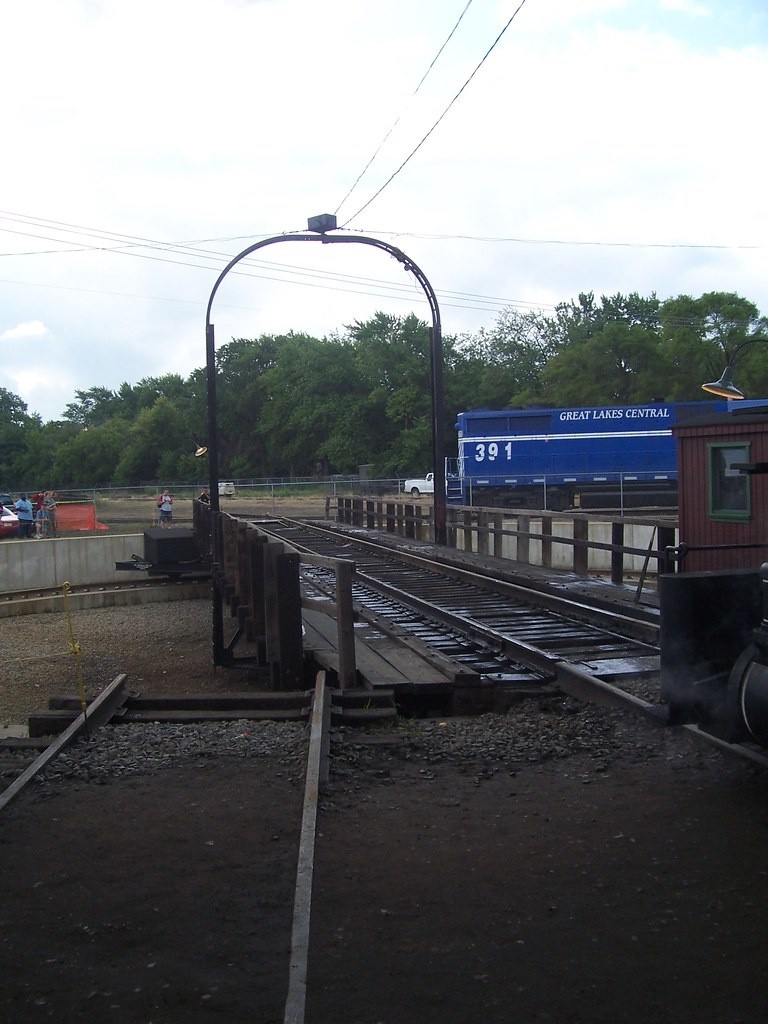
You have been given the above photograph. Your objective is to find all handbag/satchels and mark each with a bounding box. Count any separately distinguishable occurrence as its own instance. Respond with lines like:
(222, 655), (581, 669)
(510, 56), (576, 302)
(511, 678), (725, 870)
(157, 496), (162, 508)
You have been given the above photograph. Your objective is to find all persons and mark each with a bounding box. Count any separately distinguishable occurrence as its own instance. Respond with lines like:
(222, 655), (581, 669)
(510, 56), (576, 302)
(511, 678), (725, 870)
(157, 489), (173, 530)
(198, 487), (210, 505)
(32, 491), (60, 538)
(15, 493), (34, 539)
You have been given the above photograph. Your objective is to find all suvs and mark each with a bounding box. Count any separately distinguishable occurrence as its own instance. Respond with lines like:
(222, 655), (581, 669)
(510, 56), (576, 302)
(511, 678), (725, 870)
(217, 482), (236, 497)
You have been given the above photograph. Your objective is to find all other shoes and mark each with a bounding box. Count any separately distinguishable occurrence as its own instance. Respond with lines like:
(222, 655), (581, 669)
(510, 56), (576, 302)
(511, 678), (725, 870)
(168, 526), (171, 529)
(34, 534), (41, 539)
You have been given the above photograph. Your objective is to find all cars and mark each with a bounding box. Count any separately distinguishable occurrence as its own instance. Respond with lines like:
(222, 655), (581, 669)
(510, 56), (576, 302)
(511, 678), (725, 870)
(0, 491), (58, 539)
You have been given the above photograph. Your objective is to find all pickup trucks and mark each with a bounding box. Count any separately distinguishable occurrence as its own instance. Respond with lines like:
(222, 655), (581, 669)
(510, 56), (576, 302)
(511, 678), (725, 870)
(404, 472), (457, 497)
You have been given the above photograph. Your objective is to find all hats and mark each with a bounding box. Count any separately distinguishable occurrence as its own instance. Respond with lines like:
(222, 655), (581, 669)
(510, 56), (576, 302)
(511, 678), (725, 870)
(41, 502), (47, 506)
